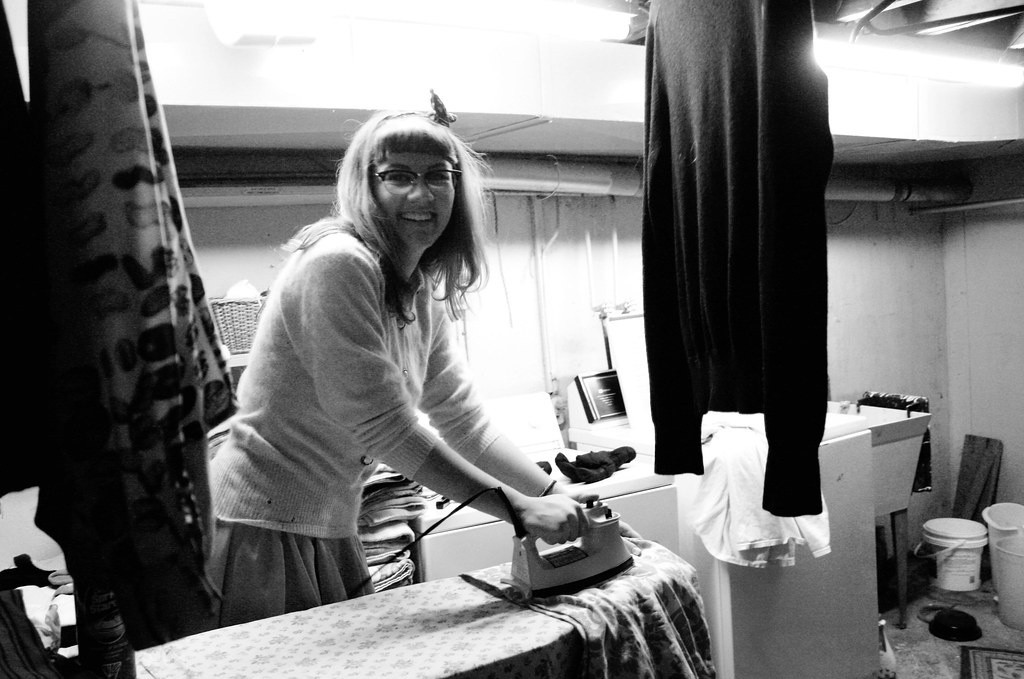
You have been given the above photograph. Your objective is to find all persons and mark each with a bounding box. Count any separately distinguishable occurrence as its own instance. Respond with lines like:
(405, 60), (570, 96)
(170, 107), (640, 638)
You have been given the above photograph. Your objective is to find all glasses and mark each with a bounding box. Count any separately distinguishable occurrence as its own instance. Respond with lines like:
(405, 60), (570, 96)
(374, 167), (462, 195)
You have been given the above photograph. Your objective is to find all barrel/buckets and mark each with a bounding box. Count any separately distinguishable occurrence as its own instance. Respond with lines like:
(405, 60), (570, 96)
(982, 502), (1024, 592)
(994, 536), (1024, 631)
(913, 518), (989, 591)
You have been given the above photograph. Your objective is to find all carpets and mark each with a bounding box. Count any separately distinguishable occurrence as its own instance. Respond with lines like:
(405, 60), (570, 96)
(959, 645), (1024, 679)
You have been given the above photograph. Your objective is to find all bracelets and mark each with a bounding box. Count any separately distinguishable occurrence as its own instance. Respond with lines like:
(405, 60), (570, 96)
(539, 479), (556, 498)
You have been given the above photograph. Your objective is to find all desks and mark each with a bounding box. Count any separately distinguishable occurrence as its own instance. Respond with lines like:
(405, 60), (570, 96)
(135, 534), (716, 679)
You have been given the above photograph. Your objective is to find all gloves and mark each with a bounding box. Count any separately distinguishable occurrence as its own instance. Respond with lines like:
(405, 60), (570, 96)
(555, 446), (636, 482)
